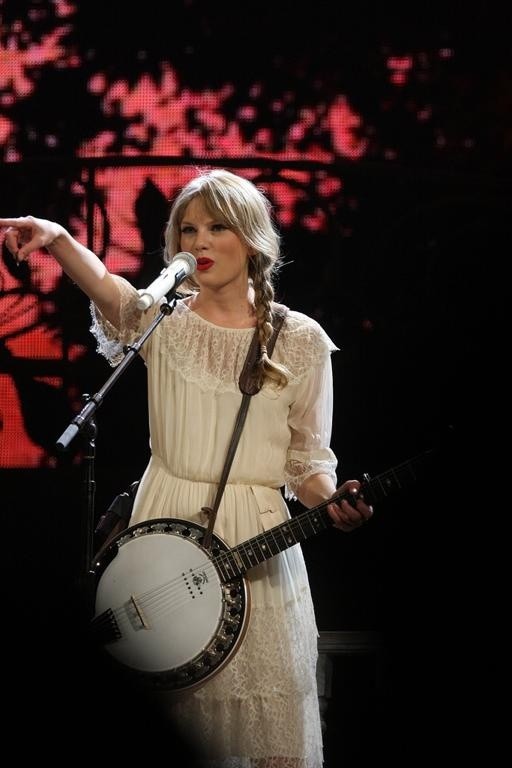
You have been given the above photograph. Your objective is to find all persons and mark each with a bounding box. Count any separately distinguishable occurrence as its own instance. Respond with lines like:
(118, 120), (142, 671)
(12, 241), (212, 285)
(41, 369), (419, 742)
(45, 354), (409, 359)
(0, 168), (376, 768)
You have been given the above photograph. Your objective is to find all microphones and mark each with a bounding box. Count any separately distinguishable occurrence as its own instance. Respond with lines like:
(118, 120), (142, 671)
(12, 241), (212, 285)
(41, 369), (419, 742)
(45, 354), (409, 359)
(137, 251), (197, 311)
